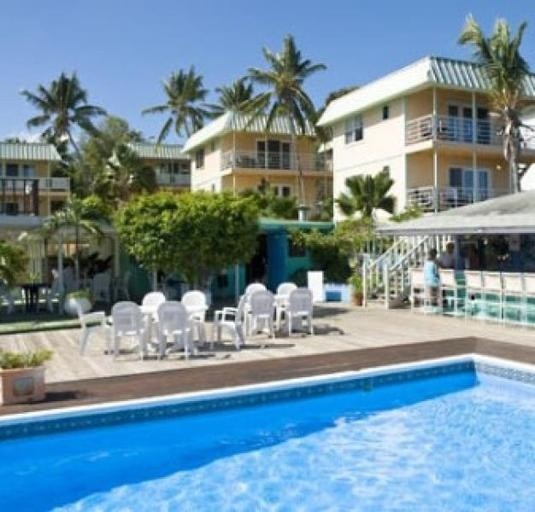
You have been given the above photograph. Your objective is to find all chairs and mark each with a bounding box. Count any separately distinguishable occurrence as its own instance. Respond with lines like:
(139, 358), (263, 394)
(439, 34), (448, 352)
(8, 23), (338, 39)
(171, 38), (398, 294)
(1, 278), (26, 315)
(68, 286), (208, 362)
(209, 280), (315, 352)
(37, 277), (64, 316)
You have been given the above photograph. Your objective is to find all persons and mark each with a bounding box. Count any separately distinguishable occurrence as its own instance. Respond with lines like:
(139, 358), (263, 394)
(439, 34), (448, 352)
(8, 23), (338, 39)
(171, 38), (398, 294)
(422, 237), (534, 308)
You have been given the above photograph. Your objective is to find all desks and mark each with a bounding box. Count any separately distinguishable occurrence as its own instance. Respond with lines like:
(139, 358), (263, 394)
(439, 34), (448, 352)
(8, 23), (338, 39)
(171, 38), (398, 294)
(16, 281), (48, 316)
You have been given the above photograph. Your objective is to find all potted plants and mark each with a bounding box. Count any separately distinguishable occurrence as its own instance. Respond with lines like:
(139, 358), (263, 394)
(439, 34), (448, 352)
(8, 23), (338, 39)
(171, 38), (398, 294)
(0, 344), (55, 405)
(349, 273), (364, 305)
